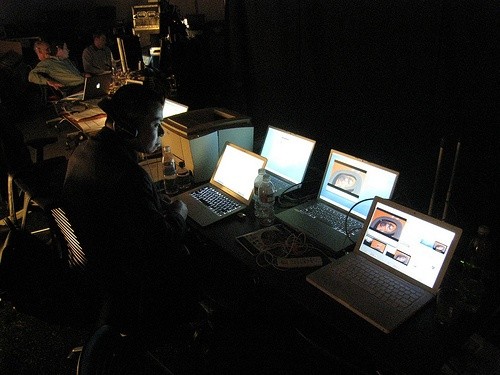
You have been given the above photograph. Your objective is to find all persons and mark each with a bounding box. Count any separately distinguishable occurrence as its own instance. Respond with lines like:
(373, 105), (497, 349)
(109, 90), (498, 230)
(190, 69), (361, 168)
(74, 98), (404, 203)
(60, 83), (189, 264)
(28, 40), (91, 90)
(82, 31), (112, 74)
(31, 41), (51, 69)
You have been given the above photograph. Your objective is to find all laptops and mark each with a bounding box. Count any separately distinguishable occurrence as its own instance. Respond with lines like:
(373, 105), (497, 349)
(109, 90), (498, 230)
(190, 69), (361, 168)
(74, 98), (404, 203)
(274, 148), (400, 253)
(66, 72), (112, 101)
(259, 125), (316, 200)
(170, 140), (268, 227)
(305, 195), (462, 335)
(162, 98), (189, 119)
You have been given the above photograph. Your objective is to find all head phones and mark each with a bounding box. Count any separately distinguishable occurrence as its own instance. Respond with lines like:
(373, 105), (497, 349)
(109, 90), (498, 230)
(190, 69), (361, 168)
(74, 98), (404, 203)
(98, 96), (139, 140)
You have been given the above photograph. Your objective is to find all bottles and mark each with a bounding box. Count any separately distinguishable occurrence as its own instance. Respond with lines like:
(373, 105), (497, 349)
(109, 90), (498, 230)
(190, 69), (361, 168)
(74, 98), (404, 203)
(164, 160), (179, 195)
(176, 160), (191, 191)
(253, 168), (267, 217)
(161, 145), (175, 173)
(464, 226), (490, 273)
(258, 174), (276, 226)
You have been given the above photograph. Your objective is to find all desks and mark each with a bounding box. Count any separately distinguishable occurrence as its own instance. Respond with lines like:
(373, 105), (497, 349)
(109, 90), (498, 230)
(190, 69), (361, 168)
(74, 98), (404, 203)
(56, 88), (500, 373)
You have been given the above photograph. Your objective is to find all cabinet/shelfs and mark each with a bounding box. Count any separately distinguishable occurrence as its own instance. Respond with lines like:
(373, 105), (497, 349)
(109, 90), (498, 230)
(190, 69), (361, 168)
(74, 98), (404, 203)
(130, 0), (163, 36)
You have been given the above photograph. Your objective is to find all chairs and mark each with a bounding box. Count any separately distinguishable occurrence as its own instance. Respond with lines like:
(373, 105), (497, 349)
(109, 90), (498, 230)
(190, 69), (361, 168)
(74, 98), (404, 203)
(8, 54), (69, 124)
(0, 125), (70, 236)
